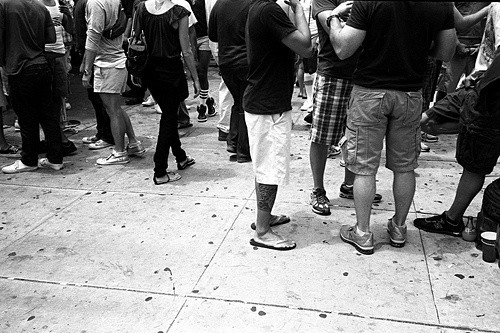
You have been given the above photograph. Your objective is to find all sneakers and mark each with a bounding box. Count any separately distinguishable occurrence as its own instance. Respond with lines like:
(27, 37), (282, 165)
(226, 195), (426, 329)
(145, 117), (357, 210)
(339, 182), (382, 202)
(310, 187), (331, 216)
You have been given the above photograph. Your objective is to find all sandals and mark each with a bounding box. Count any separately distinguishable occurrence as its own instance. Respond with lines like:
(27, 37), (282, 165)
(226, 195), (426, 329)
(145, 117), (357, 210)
(176, 156), (195, 170)
(153, 171), (182, 184)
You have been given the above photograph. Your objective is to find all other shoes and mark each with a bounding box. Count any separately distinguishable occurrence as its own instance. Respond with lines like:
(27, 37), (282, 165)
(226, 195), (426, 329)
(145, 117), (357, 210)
(205, 97), (218, 116)
(96, 149), (130, 165)
(88, 140), (113, 149)
(339, 224), (375, 255)
(327, 146), (342, 158)
(414, 211), (465, 238)
(420, 142), (430, 152)
(141, 95), (156, 106)
(38, 158), (64, 170)
(2, 160), (38, 173)
(197, 104), (208, 122)
(126, 140), (145, 154)
(177, 123), (194, 135)
(387, 215), (407, 248)
(420, 131), (437, 143)
(81, 135), (97, 143)
(0, 145), (22, 157)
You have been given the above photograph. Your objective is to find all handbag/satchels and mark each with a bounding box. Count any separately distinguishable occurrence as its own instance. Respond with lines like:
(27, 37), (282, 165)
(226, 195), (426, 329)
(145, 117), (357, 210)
(102, 6), (128, 41)
(127, 31), (149, 90)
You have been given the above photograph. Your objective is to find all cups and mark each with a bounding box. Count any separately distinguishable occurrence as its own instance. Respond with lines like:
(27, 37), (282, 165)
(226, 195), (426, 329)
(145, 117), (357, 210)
(481, 230), (498, 264)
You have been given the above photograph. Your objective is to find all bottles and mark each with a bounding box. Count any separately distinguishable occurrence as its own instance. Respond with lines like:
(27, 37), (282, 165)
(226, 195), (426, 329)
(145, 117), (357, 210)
(462, 216), (476, 242)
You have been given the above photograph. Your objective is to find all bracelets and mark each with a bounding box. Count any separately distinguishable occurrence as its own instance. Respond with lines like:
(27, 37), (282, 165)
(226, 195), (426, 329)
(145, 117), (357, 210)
(84, 70), (89, 76)
(326, 15), (340, 27)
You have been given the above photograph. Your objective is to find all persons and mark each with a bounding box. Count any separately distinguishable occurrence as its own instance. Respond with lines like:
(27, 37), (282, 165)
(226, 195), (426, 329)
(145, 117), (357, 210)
(208, 0), (500, 255)
(0, 0), (217, 185)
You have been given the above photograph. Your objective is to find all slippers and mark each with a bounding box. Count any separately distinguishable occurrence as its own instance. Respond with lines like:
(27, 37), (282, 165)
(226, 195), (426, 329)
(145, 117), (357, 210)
(251, 214), (290, 230)
(249, 233), (297, 251)
